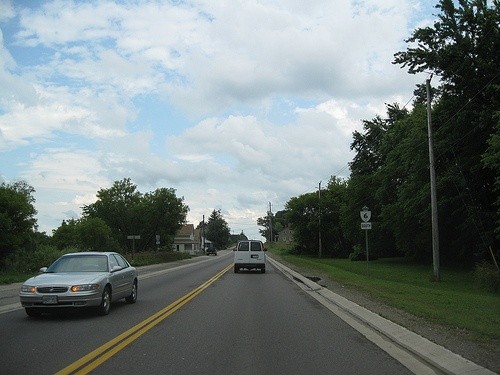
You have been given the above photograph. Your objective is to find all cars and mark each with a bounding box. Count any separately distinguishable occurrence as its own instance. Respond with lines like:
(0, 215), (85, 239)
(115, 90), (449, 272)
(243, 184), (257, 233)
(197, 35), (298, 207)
(19, 252), (139, 316)
(206, 247), (217, 256)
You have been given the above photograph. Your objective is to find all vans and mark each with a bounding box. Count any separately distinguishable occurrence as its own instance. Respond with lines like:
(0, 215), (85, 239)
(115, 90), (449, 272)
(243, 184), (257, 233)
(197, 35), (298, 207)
(233, 240), (268, 274)
